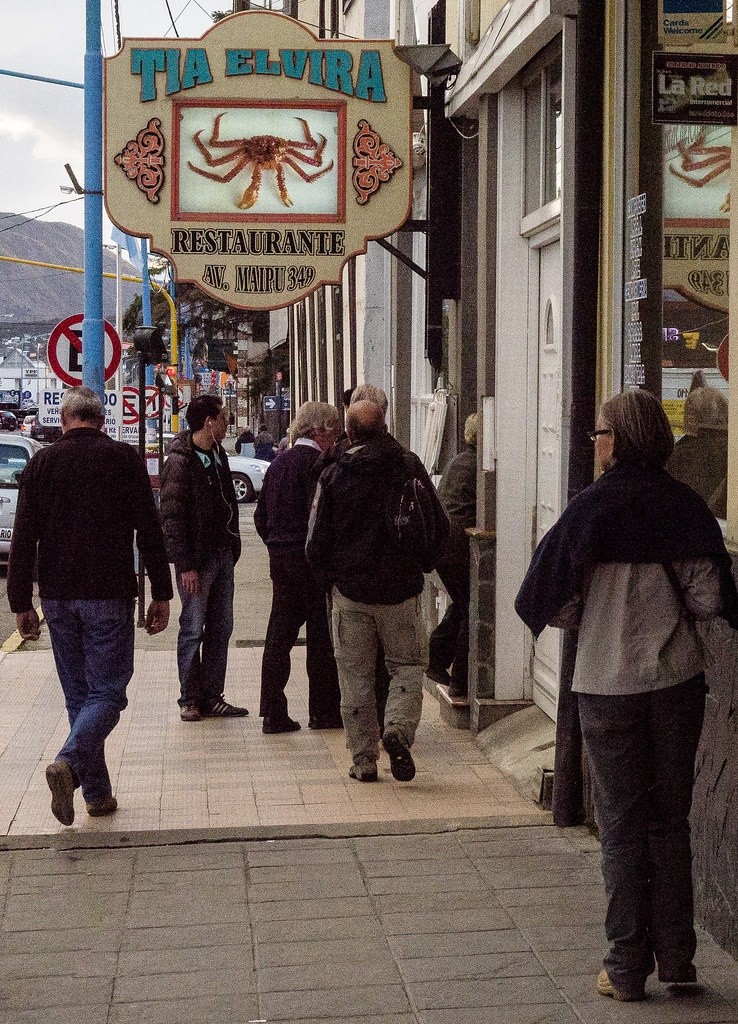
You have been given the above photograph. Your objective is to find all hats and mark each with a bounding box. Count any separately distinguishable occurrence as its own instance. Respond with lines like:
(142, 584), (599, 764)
(258, 424), (268, 432)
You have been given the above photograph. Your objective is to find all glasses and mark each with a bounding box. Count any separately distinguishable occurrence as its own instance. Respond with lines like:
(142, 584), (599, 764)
(587, 429), (610, 442)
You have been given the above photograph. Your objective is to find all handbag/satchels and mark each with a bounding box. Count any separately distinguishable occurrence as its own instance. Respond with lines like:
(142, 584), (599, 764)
(396, 452), (441, 574)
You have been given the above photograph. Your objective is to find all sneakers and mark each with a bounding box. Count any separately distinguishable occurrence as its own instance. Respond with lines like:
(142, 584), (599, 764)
(663, 982), (703, 995)
(179, 705), (201, 721)
(449, 686), (468, 697)
(308, 716), (343, 729)
(85, 797), (117, 816)
(263, 716), (301, 734)
(598, 969), (646, 1000)
(349, 765), (377, 782)
(382, 733), (416, 781)
(199, 695), (250, 718)
(46, 761), (75, 826)
(425, 664), (449, 685)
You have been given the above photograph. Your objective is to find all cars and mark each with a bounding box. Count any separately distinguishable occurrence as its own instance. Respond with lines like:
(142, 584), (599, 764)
(154, 433), (272, 503)
(0, 401), (62, 444)
(0, 434), (44, 557)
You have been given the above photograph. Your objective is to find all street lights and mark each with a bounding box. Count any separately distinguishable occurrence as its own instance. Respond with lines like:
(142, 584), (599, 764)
(104, 243), (175, 304)
(57, 184), (124, 441)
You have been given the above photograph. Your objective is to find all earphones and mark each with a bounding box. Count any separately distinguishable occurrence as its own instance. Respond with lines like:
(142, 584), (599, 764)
(209, 421), (212, 426)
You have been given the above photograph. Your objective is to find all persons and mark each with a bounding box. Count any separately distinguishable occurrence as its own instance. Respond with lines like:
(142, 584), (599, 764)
(423, 412), (477, 698)
(235, 383), (449, 784)
(663, 386), (729, 521)
(516, 388), (738, 1003)
(161, 394), (252, 722)
(7, 386), (174, 826)
